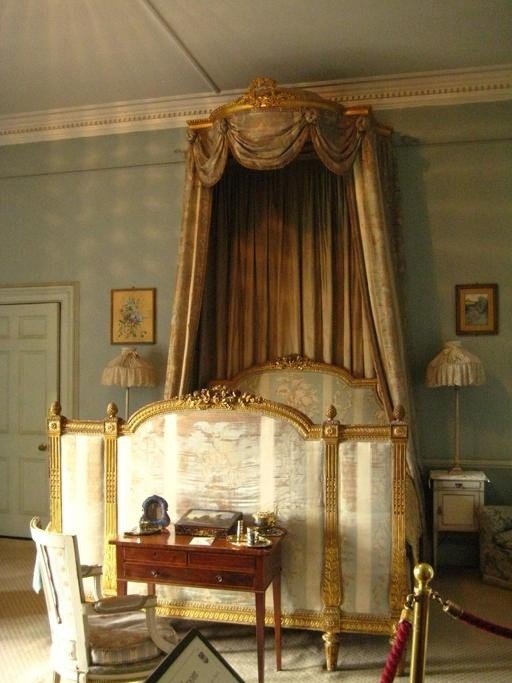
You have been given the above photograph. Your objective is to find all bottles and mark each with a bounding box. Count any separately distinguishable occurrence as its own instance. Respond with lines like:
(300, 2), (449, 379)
(236, 520), (260, 545)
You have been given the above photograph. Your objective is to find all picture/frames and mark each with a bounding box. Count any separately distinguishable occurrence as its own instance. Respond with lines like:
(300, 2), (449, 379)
(108, 288), (157, 346)
(455, 284), (497, 336)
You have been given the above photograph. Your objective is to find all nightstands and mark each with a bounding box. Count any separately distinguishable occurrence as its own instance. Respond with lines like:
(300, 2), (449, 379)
(429, 469), (485, 571)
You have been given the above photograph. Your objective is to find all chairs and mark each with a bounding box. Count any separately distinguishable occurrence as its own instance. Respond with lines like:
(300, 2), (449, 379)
(479, 506), (512, 589)
(29, 515), (178, 683)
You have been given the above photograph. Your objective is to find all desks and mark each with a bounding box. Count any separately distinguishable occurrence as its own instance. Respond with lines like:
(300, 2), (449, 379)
(110, 524), (287, 682)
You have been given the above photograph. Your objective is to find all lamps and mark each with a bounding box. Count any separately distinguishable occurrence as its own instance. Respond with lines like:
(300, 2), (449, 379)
(426, 339), (487, 473)
(101, 347), (161, 422)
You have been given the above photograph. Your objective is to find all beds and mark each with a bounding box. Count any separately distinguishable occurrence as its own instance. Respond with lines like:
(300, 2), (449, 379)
(47, 355), (410, 672)
